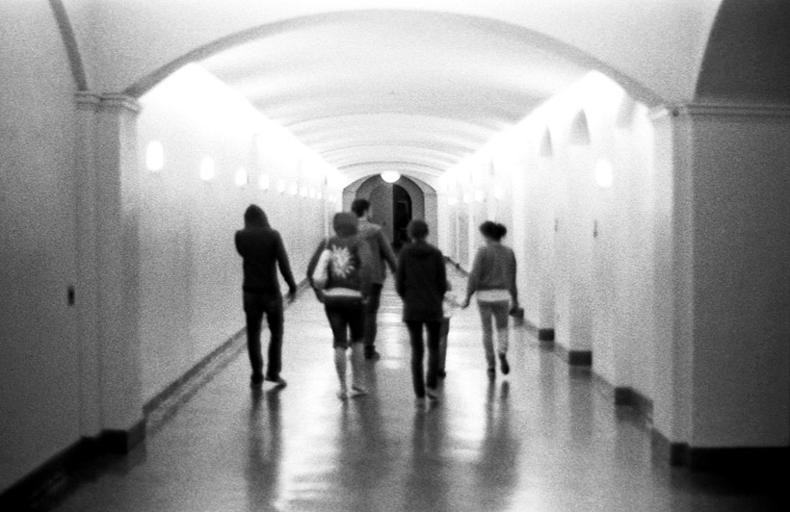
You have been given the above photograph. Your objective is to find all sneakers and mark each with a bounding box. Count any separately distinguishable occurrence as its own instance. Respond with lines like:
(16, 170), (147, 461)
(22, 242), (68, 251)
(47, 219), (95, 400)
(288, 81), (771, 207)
(424, 388), (441, 400)
(265, 372), (286, 386)
(250, 378), (262, 389)
(499, 352), (509, 375)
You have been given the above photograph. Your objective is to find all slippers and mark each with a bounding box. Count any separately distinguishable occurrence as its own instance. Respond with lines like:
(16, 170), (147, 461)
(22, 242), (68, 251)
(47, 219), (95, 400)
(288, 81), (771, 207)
(350, 384), (370, 398)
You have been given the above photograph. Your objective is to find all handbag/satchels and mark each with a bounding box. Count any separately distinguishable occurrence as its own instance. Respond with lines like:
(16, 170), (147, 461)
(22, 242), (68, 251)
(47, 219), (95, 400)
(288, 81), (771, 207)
(311, 248), (332, 290)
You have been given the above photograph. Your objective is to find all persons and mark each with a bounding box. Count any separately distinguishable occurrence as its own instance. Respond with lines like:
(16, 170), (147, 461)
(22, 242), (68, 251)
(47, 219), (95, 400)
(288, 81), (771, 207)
(307, 211), (373, 398)
(235, 204), (296, 387)
(394, 220), (446, 405)
(351, 198), (397, 360)
(460, 221), (518, 374)
(439, 280), (462, 377)
